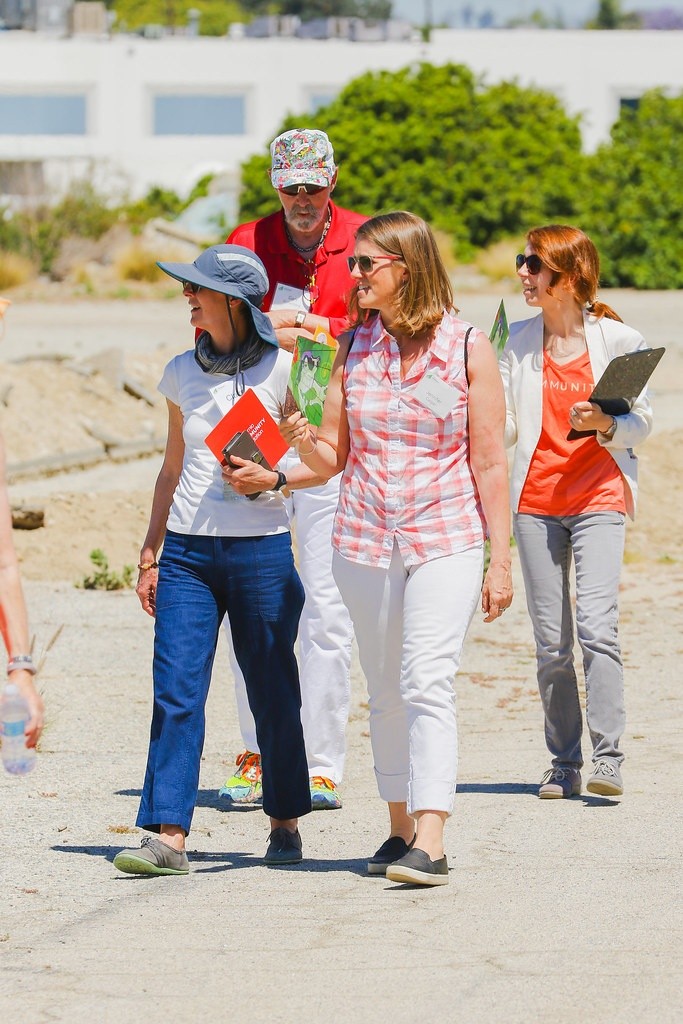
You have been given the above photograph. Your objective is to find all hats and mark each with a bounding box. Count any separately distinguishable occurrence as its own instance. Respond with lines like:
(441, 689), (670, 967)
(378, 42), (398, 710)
(156, 244), (280, 350)
(270, 128), (335, 189)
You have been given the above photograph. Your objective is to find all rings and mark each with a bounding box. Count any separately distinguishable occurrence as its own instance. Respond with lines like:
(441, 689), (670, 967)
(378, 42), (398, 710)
(290, 431), (295, 438)
(227, 481), (230, 486)
(499, 607), (506, 612)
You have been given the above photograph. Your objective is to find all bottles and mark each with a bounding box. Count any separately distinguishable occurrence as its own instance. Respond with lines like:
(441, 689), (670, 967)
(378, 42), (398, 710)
(0, 681), (37, 775)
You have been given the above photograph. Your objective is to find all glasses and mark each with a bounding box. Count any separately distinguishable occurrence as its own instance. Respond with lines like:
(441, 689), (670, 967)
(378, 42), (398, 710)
(515, 255), (545, 275)
(279, 184), (326, 195)
(347, 255), (402, 272)
(183, 280), (204, 293)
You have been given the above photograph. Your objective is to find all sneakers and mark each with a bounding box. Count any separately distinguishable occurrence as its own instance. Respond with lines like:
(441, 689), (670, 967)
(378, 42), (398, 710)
(309, 776), (342, 809)
(218, 751), (263, 807)
(586, 759), (623, 795)
(539, 766), (582, 798)
(113, 835), (190, 873)
(263, 827), (303, 864)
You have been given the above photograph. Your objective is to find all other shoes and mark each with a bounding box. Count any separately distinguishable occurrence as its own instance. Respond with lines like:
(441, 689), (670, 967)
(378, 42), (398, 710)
(367, 833), (417, 874)
(386, 848), (449, 885)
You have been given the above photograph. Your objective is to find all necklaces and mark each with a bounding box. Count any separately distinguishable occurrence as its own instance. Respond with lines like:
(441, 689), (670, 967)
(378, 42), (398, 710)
(285, 208), (332, 252)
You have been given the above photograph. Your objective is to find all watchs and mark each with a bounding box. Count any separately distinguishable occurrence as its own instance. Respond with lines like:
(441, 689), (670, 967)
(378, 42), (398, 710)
(599, 417), (617, 438)
(293, 311), (307, 328)
(271, 470), (287, 491)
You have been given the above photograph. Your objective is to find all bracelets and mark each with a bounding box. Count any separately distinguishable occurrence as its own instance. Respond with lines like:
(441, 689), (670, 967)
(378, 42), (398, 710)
(137, 561), (158, 570)
(7, 656), (37, 677)
(296, 446), (317, 455)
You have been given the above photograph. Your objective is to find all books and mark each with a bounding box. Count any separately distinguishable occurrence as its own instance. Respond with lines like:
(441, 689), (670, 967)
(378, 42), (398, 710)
(222, 430), (274, 501)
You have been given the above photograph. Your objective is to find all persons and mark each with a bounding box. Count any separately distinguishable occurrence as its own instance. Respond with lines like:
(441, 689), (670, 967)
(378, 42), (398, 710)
(280, 210), (514, 887)
(113, 244), (326, 877)
(195, 129), (377, 810)
(0, 431), (44, 747)
(493, 223), (654, 801)
